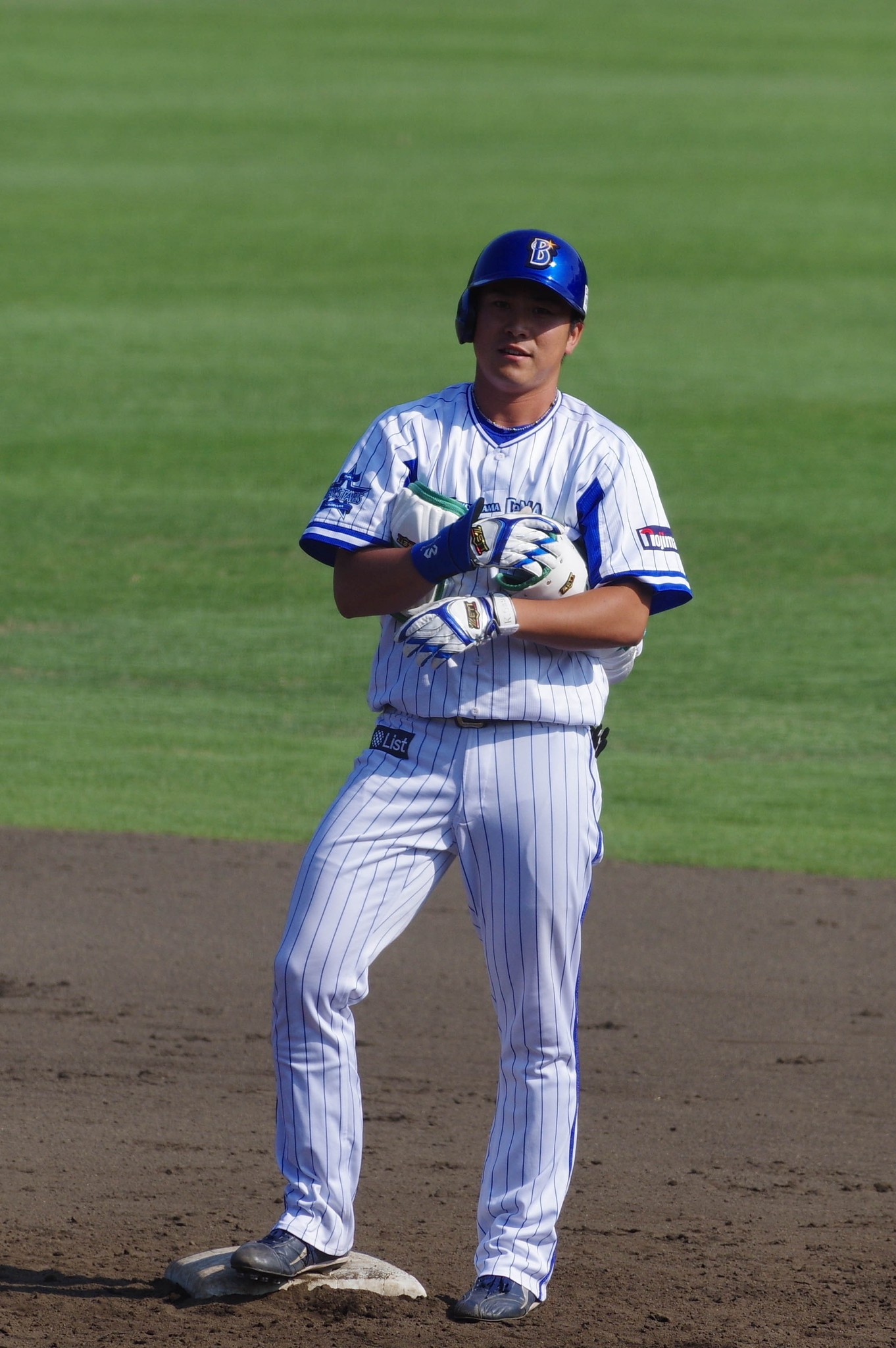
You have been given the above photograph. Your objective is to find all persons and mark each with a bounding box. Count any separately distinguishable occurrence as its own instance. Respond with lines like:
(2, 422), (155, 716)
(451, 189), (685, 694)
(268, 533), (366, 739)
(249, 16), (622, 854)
(232, 229), (692, 1322)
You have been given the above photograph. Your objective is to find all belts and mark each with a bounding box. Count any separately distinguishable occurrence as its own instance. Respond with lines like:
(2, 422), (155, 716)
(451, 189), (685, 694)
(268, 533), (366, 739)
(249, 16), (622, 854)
(418, 715), (532, 728)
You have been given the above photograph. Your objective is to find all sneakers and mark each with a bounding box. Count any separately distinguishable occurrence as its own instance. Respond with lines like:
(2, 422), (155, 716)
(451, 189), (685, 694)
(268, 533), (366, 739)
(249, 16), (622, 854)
(231, 1229), (350, 1277)
(451, 1275), (541, 1321)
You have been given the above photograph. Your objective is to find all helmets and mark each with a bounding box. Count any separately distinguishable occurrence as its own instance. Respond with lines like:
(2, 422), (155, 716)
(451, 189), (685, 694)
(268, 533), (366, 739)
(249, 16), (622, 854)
(456, 228), (588, 344)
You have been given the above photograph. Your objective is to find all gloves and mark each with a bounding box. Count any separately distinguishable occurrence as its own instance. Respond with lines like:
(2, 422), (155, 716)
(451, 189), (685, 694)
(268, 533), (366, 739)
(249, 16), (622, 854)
(468, 506), (565, 578)
(394, 593), (519, 670)
